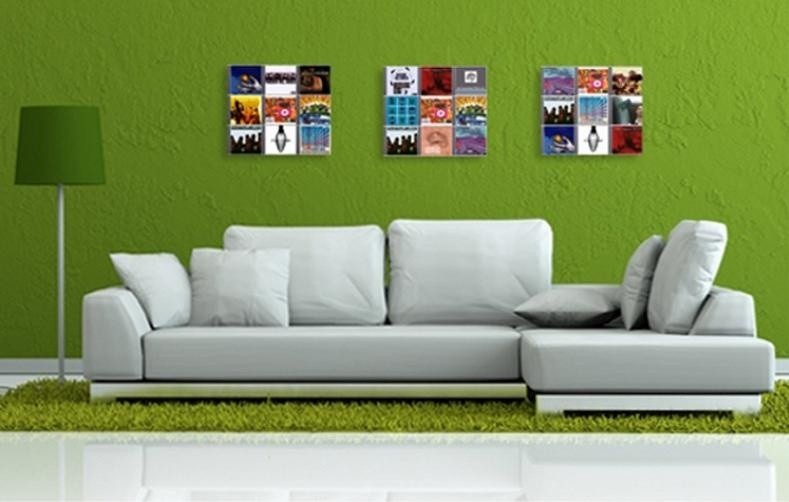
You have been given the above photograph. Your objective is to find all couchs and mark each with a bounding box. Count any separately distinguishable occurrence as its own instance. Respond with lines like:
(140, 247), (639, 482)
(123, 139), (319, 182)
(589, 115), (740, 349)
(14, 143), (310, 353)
(78, 218), (776, 421)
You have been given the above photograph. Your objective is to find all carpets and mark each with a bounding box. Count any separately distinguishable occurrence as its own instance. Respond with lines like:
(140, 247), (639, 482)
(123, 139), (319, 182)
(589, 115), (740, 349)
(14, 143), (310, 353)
(0, 376), (788, 433)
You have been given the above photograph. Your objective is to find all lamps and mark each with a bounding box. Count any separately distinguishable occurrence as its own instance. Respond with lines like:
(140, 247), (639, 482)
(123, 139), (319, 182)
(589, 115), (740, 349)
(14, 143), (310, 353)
(13, 105), (107, 381)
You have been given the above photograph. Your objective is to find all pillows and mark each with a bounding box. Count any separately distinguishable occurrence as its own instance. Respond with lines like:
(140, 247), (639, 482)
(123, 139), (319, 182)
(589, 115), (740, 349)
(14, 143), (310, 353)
(514, 219), (663, 332)
(107, 246), (296, 331)
